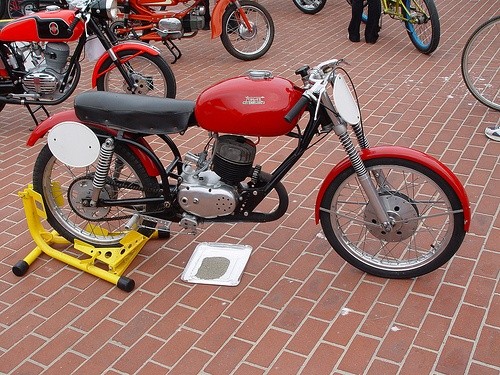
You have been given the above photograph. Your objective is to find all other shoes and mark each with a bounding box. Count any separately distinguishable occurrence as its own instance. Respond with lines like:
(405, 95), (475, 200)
(365, 34), (379, 42)
(349, 33), (360, 42)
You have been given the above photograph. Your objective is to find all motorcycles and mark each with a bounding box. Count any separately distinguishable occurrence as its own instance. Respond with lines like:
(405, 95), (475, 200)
(0, 0), (176, 131)
(86, 0), (275, 62)
(10, 58), (471, 292)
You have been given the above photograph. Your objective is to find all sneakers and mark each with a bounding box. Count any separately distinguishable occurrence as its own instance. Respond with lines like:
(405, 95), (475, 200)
(484, 125), (500, 142)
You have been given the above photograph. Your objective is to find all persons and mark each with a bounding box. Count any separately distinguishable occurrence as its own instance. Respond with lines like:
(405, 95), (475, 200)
(347, 0), (384, 44)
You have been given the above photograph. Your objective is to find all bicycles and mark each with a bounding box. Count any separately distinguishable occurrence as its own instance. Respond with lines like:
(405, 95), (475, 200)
(345, 0), (441, 55)
